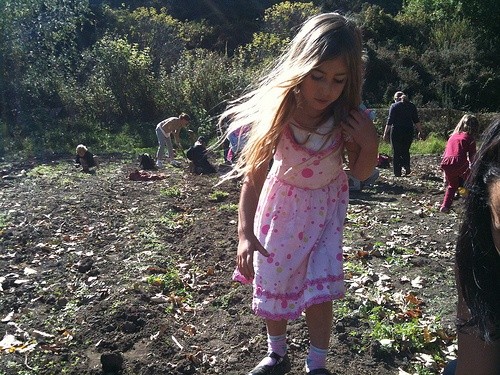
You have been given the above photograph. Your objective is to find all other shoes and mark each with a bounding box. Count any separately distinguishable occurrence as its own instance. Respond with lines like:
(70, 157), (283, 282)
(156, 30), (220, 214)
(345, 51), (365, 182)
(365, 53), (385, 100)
(440, 205), (449, 213)
(251, 351), (292, 375)
(170, 160), (181, 164)
(303, 366), (337, 375)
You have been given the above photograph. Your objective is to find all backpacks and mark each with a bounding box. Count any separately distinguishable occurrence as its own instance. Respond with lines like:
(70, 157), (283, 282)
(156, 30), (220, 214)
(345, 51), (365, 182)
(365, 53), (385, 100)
(139, 153), (158, 170)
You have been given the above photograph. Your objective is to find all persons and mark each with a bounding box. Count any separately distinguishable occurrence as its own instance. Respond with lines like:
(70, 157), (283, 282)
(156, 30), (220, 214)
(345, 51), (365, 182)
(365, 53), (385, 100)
(156, 113), (190, 167)
(440, 114), (480, 213)
(76, 144), (101, 173)
(210, 12), (379, 375)
(383, 91), (422, 177)
(187, 136), (206, 175)
(443, 119), (500, 375)
